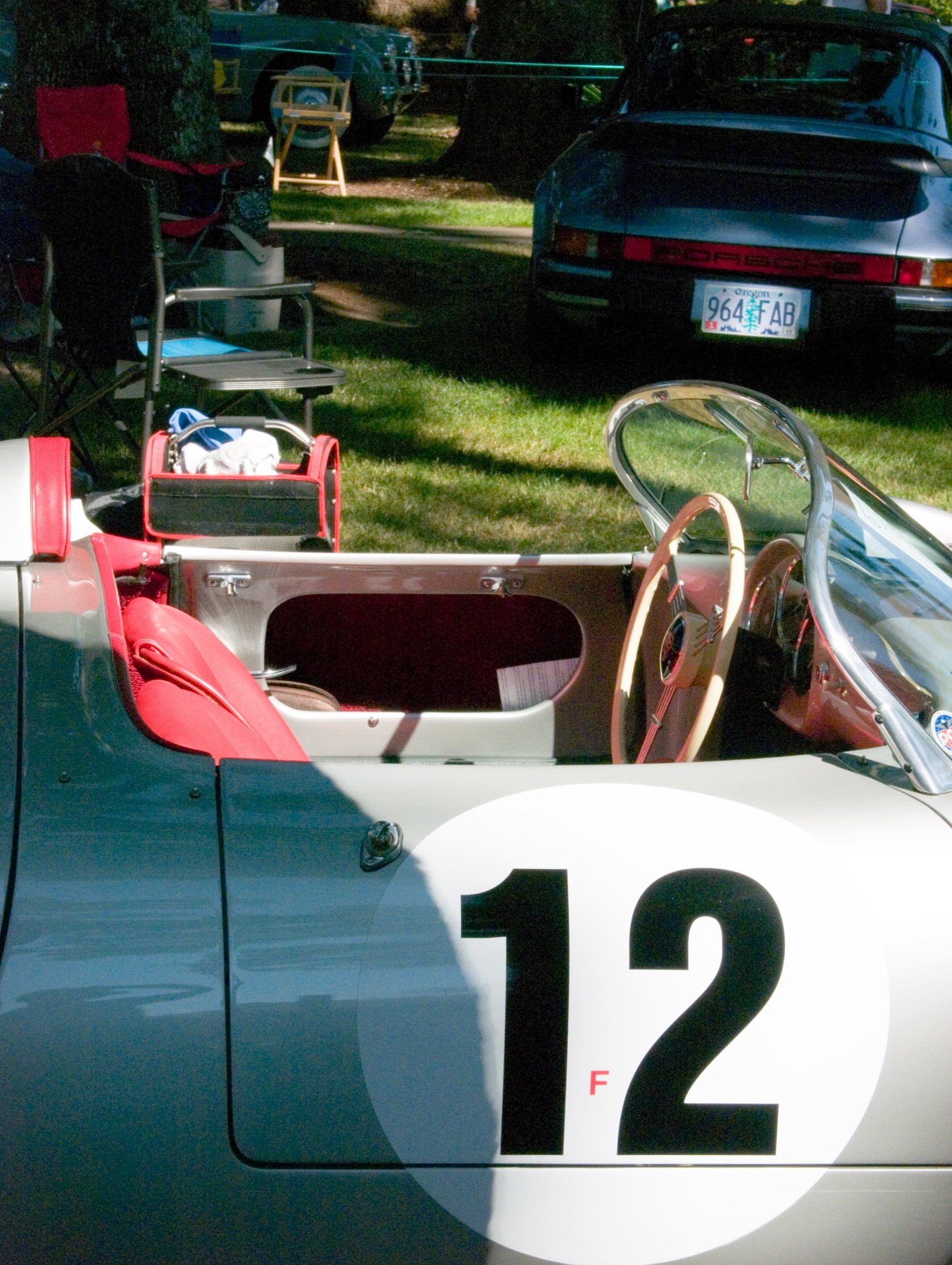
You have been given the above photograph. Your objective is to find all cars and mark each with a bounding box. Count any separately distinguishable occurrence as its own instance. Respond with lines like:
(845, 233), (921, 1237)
(0, 1), (423, 168)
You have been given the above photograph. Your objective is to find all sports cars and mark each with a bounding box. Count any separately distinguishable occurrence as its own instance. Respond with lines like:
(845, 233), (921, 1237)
(528, 0), (951, 384)
(0, 378), (951, 1263)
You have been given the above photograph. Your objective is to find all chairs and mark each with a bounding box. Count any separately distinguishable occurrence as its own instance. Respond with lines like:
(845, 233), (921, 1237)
(274, 38), (357, 197)
(33, 151), (315, 457)
(209, 59), (261, 163)
(125, 595), (309, 761)
(0, 145), (146, 467)
(36, 84), (271, 335)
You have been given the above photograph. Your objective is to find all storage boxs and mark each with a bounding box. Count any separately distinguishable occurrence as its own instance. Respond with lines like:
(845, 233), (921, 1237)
(169, 232), (287, 335)
